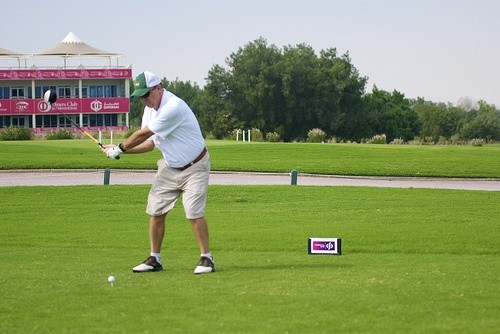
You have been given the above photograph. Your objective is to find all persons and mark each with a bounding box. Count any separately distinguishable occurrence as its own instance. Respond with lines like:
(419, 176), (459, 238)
(100, 70), (215, 274)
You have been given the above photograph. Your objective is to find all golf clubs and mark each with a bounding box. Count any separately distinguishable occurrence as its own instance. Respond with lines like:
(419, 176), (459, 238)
(44, 89), (121, 160)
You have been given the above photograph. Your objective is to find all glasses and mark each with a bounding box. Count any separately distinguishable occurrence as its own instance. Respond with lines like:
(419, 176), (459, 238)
(140, 85), (157, 98)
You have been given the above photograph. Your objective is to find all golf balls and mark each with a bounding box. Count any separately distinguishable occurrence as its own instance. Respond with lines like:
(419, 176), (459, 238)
(108, 275), (115, 282)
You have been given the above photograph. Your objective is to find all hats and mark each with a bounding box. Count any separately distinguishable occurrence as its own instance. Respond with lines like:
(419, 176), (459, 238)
(131, 71), (161, 96)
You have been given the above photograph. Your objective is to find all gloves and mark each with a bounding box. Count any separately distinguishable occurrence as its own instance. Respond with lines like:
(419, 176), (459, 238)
(106, 143), (126, 159)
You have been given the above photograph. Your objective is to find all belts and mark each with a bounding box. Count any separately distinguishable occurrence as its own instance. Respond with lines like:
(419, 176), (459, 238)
(178, 147), (207, 171)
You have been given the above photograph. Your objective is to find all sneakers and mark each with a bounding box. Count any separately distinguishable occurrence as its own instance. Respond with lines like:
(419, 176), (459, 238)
(194, 256), (215, 274)
(133, 256), (163, 273)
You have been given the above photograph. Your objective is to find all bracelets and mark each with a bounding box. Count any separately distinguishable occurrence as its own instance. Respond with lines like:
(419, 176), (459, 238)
(119, 144), (125, 153)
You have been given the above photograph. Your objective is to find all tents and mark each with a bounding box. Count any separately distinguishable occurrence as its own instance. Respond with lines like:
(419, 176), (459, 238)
(0, 46), (31, 70)
(33, 31), (124, 68)
